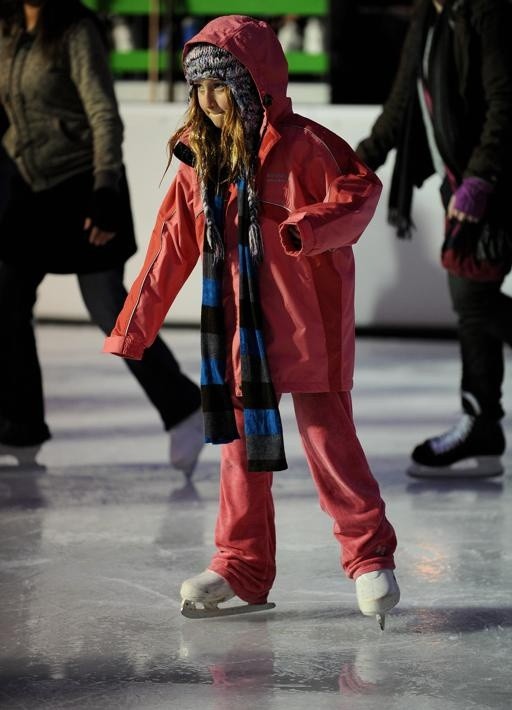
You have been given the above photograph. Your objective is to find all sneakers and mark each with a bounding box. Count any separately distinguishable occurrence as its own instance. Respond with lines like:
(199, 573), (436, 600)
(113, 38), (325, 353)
(180, 568), (234, 603)
(412, 415), (505, 466)
(169, 409), (203, 466)
(355, 570), (399, 615)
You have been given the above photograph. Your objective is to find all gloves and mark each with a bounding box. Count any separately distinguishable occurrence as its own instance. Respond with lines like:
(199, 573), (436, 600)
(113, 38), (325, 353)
(441, 177), (489, 259)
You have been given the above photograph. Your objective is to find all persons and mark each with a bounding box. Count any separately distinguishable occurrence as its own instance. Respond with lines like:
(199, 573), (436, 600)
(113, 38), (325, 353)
(105, 13), (406, 618)
(0, 0), (217, 481)
(351, 0), (510, 467)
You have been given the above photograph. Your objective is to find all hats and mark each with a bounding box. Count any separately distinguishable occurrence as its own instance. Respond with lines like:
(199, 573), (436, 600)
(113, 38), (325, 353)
(187, 43), (264, 129)
